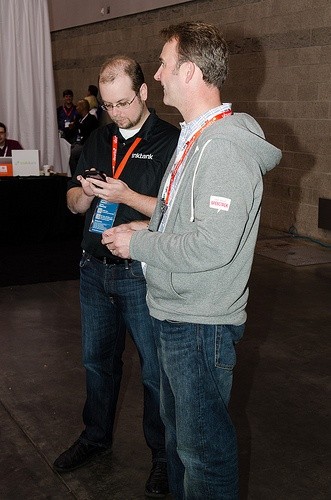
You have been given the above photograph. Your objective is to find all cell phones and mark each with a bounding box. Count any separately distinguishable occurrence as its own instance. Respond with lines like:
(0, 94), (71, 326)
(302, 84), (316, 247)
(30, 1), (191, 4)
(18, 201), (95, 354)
(84, 168), (107, 189)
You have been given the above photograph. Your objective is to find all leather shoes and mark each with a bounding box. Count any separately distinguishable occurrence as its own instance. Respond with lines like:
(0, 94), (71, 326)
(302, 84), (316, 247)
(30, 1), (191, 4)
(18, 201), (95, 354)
(145, 452), (171, 497)
(53, 431), (112, 472)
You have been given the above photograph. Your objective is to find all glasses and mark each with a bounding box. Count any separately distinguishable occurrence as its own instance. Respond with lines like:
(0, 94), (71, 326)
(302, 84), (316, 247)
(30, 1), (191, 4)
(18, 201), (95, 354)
(100, 86), (140, 111)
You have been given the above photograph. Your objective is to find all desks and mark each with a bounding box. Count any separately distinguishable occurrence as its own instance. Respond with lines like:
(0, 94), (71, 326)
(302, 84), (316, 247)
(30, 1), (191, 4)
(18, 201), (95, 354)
(0, 176), (84, 287)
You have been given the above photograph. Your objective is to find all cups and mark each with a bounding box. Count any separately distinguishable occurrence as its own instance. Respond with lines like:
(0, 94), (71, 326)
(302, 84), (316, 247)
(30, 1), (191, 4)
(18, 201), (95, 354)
(43, 165), (51, 176)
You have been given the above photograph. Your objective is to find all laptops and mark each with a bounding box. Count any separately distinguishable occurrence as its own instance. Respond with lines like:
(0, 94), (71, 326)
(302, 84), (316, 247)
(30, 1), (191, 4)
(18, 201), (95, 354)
(0, 157), (12, 165)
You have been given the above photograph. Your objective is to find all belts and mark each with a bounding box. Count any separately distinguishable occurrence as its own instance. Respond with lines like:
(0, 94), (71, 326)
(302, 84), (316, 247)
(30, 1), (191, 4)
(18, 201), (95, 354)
(86, 250), (132, 265)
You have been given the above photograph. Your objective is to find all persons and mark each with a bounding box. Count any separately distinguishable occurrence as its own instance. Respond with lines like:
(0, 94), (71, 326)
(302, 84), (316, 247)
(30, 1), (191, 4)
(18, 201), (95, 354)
(100, 20), (282, 500)
(68, 97), (97, 177)
(56, 89), (80, 144)
(0, 123), (23, 157)
(49, 51), (179, 475)
(84, 85), (98, 109)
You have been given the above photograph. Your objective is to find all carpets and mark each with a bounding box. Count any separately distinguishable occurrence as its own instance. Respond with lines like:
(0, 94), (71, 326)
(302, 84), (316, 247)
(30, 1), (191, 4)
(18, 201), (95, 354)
(255, 239), (331, 266)
